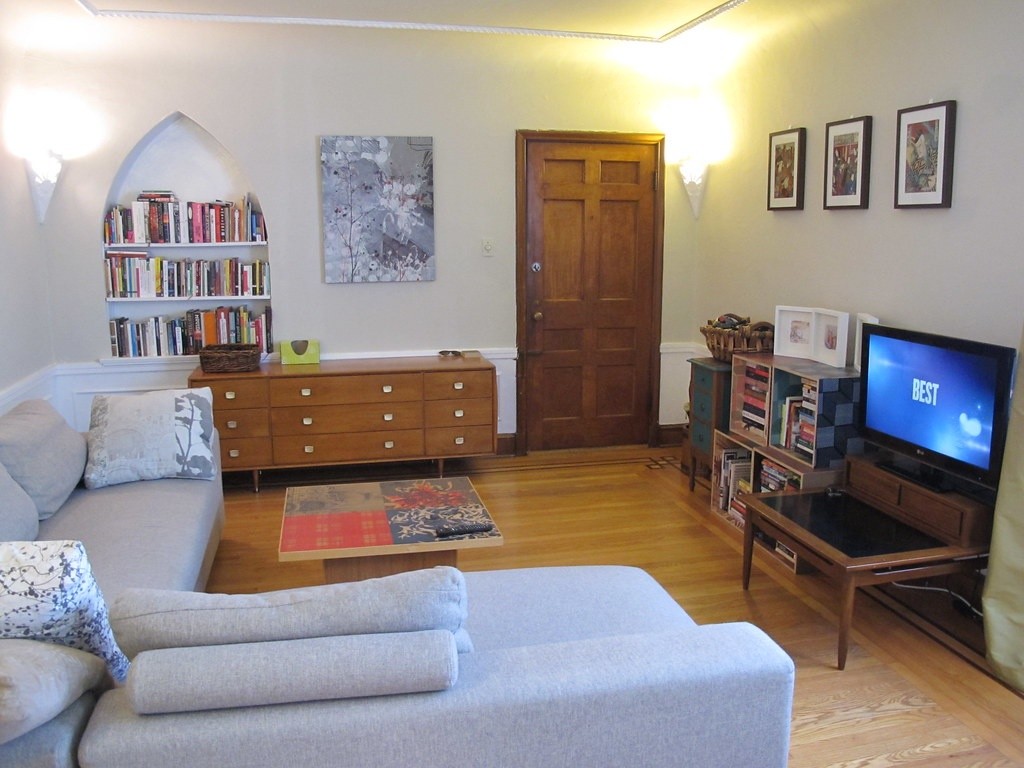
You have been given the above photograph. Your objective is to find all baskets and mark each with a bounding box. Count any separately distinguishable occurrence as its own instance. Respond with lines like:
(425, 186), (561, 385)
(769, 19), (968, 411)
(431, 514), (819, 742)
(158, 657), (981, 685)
(699, 313), (775, 364)
(198, 343), (261, 373)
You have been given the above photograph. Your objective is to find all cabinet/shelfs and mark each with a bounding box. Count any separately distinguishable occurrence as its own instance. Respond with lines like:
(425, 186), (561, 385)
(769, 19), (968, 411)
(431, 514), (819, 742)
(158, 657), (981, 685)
(684, 355), (731, 493)
(190, 356), (497, 493)
(709, 352), (862, 578)
(108, 242), (272, 358)
(730, 457), (993, 671)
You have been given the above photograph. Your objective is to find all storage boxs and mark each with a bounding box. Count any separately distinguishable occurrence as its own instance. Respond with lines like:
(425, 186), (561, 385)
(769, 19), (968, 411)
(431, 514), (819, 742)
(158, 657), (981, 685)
(853, 313), (879, 372)
(774, 306), (813, 359)
(811, 308), (850, 368)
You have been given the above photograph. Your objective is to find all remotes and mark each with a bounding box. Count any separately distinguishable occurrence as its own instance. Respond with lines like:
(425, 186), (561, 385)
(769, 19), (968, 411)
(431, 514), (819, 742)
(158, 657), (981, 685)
(435, 524), (492, 537)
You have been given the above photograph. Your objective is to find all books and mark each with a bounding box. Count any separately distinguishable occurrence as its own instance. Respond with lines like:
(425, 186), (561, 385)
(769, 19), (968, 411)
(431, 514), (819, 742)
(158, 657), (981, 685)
(109, 305), (272, 358)
(741, 360), (768, 438)
(779, 378), (817, 464)
(719, 447), (801, 564)
(104, 190), (265, 244)
(104, 251), (270, 298)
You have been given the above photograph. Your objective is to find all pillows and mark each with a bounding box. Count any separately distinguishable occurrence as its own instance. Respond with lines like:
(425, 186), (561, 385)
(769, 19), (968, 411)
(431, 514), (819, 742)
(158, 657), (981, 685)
(84, 385), (215, 490)
(0, 538), (130, 686)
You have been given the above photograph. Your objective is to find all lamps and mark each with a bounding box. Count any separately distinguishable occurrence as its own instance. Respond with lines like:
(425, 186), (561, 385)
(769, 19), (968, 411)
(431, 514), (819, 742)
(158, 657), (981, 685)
(679, 160), (708, 222)
(26, 154), (64, 225)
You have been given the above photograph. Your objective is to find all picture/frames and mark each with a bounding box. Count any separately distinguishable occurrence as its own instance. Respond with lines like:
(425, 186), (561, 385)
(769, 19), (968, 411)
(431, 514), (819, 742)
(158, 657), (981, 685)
(822, 115), (872, 210)
(766, 126), (806, 212)
(894, 99), (957, 210)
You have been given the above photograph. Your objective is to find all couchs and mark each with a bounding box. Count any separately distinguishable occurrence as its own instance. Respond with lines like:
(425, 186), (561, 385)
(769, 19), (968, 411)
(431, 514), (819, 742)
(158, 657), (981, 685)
(79, 565), (795, 768)
(0, 397), (228, 768)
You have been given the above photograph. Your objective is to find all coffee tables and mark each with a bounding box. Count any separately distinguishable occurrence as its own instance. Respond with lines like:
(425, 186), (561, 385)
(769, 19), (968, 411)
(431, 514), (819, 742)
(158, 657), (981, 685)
(282, 476), (504, 584)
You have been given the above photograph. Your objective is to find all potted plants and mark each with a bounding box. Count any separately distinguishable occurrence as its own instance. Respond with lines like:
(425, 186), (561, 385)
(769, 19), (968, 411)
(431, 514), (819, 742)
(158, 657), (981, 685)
(679, 402), (712, 477)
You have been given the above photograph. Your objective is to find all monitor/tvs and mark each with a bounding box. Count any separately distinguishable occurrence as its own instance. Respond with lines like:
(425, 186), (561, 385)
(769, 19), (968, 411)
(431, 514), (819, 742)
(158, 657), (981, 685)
(860, 322), (1017, 509)
